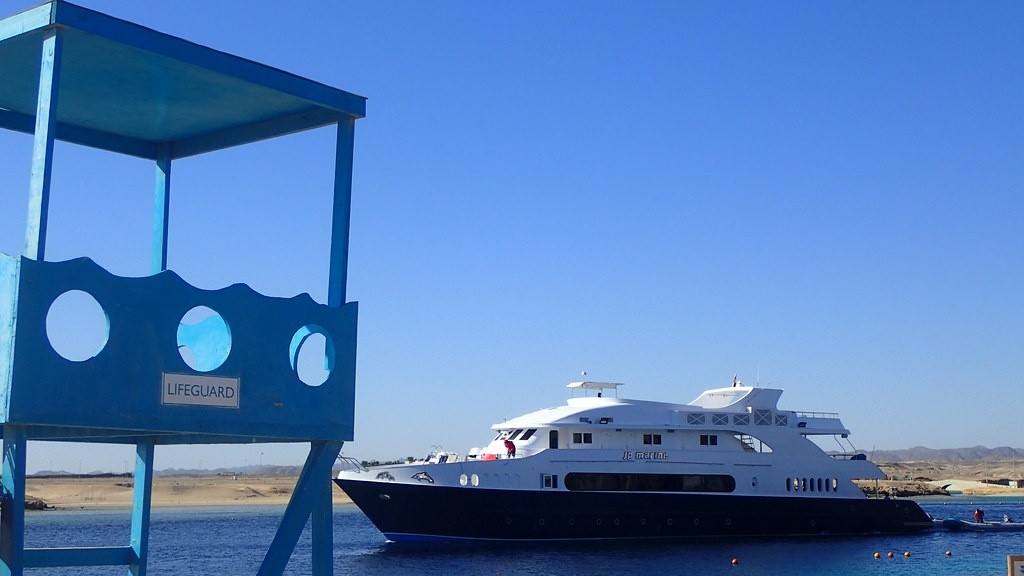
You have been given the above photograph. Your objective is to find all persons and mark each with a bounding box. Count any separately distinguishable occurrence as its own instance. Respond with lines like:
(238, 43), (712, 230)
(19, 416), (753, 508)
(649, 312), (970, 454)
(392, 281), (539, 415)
(884, 492), (890, 499)
(973, 509), (984, 523)
(504, 440), (516, 457)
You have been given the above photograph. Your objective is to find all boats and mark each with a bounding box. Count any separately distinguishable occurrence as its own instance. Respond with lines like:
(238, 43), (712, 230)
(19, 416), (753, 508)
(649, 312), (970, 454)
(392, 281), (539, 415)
(333, 371), (937, 542)
(932, 516), (1024, 531)
